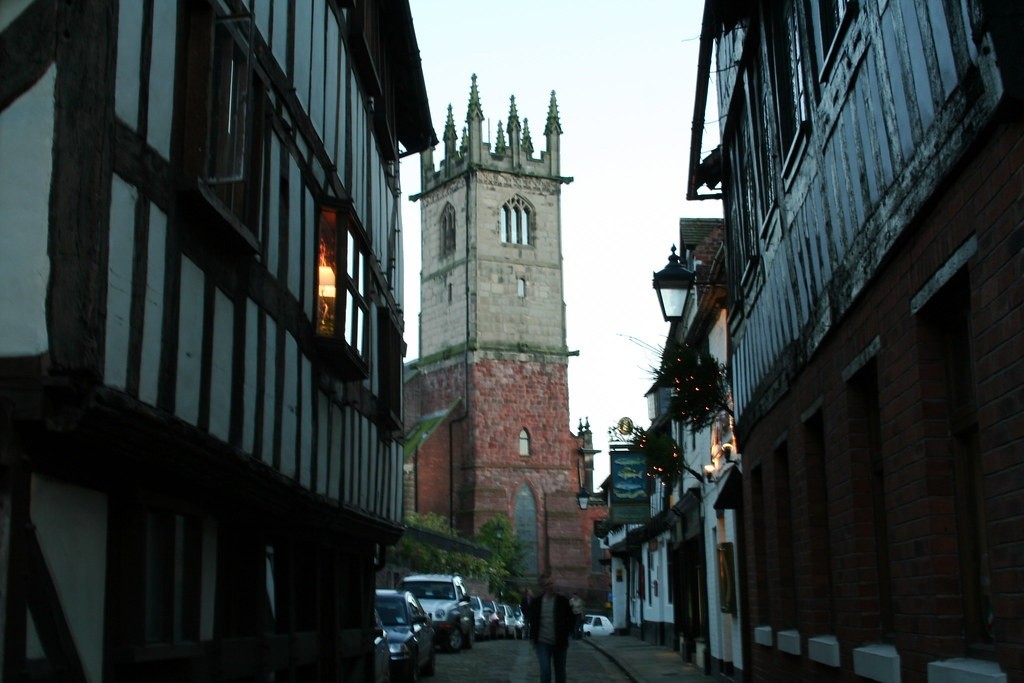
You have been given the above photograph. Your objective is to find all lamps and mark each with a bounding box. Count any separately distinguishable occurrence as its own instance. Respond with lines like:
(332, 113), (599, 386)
(652, 246), (729, 322)
(576, 484), (590, 510)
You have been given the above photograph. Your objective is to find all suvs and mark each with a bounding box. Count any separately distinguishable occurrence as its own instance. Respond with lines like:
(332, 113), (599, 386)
(396, 572), (476, 655)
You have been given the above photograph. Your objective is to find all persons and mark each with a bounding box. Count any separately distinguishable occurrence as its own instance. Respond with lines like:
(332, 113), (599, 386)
(521, 572), (586, 683)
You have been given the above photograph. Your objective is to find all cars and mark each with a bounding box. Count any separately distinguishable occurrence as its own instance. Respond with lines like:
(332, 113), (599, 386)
(482, 598), (506, 641)
(577, 614), (616, 639)
(496, 603), (519, 641)
(468, 595), (486, 642)
(373, 589), (437, 683)
(375, 608), (393, 683)
(511, 604), (533, 641)
